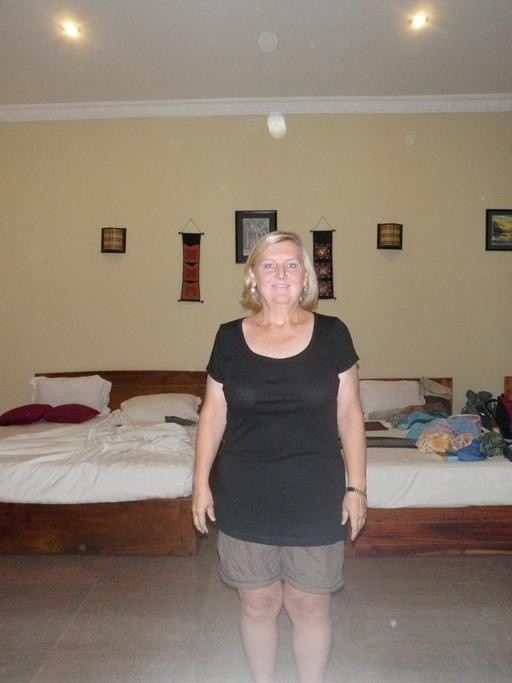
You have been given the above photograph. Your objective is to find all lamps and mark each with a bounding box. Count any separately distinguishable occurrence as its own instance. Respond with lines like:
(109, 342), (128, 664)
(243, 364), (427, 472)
(376, 221), (403, 250)
(99, 226), (127, 253)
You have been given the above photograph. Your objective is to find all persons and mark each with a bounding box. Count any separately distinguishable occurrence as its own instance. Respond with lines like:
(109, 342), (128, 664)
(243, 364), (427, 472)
(189, 229), (370, 683)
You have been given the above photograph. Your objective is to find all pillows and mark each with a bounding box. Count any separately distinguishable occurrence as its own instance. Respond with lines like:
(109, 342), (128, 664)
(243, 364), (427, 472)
(1, 374), (202, 426)
(356, 380), (425, 422)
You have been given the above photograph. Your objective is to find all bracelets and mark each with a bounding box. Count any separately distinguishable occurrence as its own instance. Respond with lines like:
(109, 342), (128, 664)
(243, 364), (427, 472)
(345, 485), (369, 499)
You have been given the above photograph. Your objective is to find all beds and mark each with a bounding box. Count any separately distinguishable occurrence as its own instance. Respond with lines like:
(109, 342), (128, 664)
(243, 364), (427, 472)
(340, 376), (511, 561)
(1, 368), (207, 557)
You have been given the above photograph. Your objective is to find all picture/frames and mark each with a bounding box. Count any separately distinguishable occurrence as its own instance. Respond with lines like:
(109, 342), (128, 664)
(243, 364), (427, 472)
(484, 208), (512, 251)
(233, 207), (279, 264)
